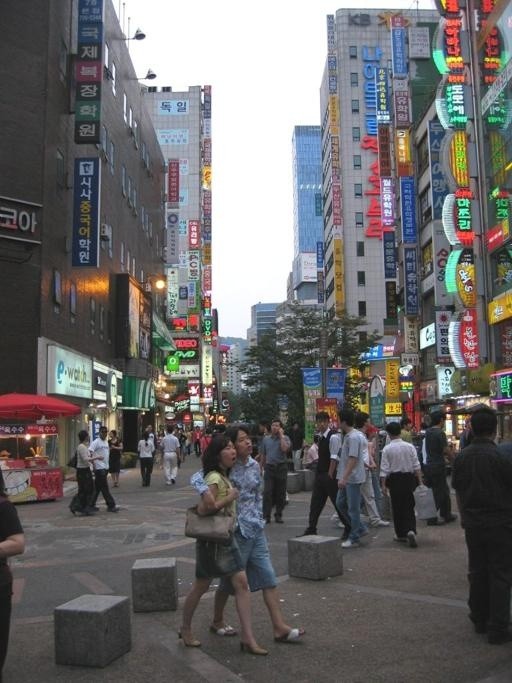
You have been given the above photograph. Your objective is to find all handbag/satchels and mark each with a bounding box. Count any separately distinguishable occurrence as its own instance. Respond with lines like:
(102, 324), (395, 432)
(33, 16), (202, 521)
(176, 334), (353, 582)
(184, 473), (235, 545)
(413, 485), (439, 521)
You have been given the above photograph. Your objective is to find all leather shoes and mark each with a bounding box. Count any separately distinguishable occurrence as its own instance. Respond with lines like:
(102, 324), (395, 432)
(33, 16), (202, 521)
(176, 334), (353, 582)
(297, 530), (317, 536)
(108, 507), (119, 511)
(276, 519), (283, 522)
(265, 519), (270, 523)
(94, 505), (99, 511)
(338, 527), (350, 541)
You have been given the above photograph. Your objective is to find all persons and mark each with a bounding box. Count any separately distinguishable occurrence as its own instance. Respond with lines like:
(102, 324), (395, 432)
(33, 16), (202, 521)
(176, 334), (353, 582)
(178, 435), (268, 655)
(295, 411), (351, 539)
(190, 426), (306, 640)
(305, 435), (322, 471)
(355, 411), (391, 521)
(380, 422), (422, 548)
(452, 409), (512, 645)
(174, 427), (187, 470)
(90, 427), (120, 512)
(330, 412), (390, 526)
(458, 405), (491, 450)
(425, 411), (457, 526)
(399, 418), (414, 443)
(194, 426), (203, 454)
(213, 424), (227, 433)
(0, 463), (26, 683)
(161, 427), (182, 484)
(145, 425), (157, 459)
(422, 423), (431, 476)
(254, 421), (291, 504)
(68, 431), (104, 517)
(107, 430), (124, 488)
(157, 424), (196, 457)
(1, 450), (11, 458)
(258, 420), (292, 525)
(138, 432), (156, 487)
(200, 430), (213, 460)
(290, 421), (308, 472)
(335, 410), (370, 548)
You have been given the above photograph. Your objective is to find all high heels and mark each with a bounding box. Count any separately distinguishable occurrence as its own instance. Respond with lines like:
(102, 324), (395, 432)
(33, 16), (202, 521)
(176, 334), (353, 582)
(68, 504), (76, 515)
(177, 627), (201, 646)
(83, 506), (97, 518)
(240, 641), (268, 655)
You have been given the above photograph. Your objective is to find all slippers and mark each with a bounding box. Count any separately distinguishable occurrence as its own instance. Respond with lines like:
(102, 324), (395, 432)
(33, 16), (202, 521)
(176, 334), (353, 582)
(209, 623), (238, 636)
(273, 627), (307, 642)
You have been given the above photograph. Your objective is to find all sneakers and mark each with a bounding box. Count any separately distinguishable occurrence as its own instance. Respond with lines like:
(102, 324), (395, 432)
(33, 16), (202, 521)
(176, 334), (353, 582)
(407, 530), (417, 548)
(444, 514), (458, 522)
(331, 512), (344, 529)
(488, 627), (512, 643)
(392, 533), (408, 541)
(340, 539), (360, 547)
(165, 481), (171, 486)
(472, 619), (489, 634)
(171, 477), (175, 484)
(359, 528), (370, 537)
(369, 519), (391, 527)
